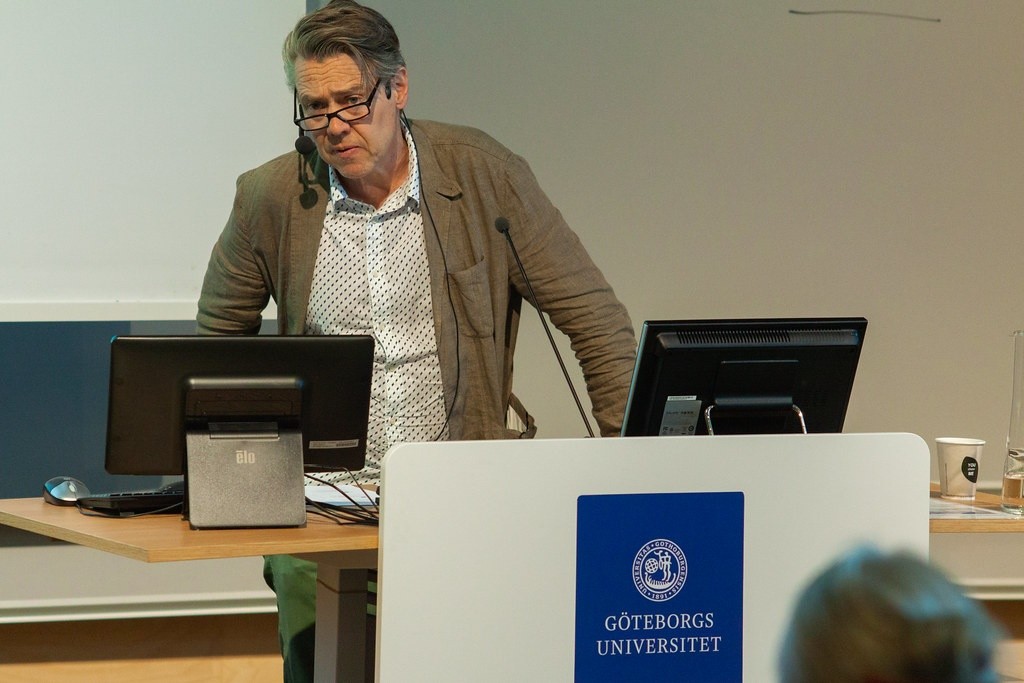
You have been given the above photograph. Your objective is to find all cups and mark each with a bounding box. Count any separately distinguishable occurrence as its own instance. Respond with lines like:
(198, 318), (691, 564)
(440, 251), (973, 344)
(935, 437), (986, 502)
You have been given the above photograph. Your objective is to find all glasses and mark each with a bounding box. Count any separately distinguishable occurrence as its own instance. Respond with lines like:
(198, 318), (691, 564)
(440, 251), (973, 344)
(293, 77), (382, 131)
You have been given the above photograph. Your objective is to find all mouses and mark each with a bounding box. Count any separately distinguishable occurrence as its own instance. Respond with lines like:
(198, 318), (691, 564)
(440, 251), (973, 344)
(43, 475), (91, 505)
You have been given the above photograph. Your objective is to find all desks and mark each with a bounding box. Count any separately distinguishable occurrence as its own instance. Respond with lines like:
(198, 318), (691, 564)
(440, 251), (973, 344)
(0, 471), (1024, 683)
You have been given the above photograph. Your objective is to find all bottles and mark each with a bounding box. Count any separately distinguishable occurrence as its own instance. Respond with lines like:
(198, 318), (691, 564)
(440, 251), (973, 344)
(1000, 330), (1024, 516)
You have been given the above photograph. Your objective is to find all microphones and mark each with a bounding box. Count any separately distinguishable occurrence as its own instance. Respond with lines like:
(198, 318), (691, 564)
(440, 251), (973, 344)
(495, 216), (594, 438)
(295, 128), (316, 155)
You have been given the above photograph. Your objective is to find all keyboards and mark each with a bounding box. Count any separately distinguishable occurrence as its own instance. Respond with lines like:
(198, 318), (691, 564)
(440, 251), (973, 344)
(76, 489), (183, 517)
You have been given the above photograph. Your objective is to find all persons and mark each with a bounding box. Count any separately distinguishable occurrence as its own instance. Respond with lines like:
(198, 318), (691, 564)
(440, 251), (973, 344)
(778, 553), (1003, 683)
(194, 0), (635, 683)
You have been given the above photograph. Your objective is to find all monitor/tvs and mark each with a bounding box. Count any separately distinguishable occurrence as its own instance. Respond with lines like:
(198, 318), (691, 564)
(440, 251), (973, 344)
(619, 315), (869, 438)
(108, 333), (377, 528)
